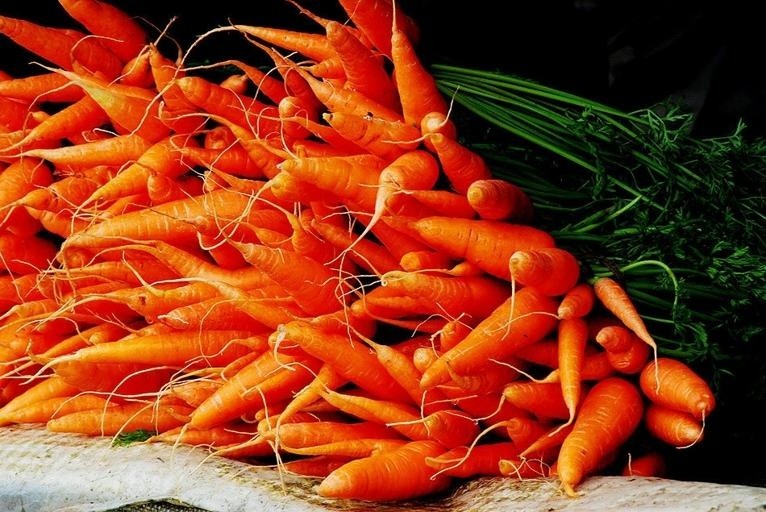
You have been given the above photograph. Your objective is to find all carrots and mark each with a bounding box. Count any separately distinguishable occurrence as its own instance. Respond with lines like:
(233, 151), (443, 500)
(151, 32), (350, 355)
(0, 0), (718, 501)
